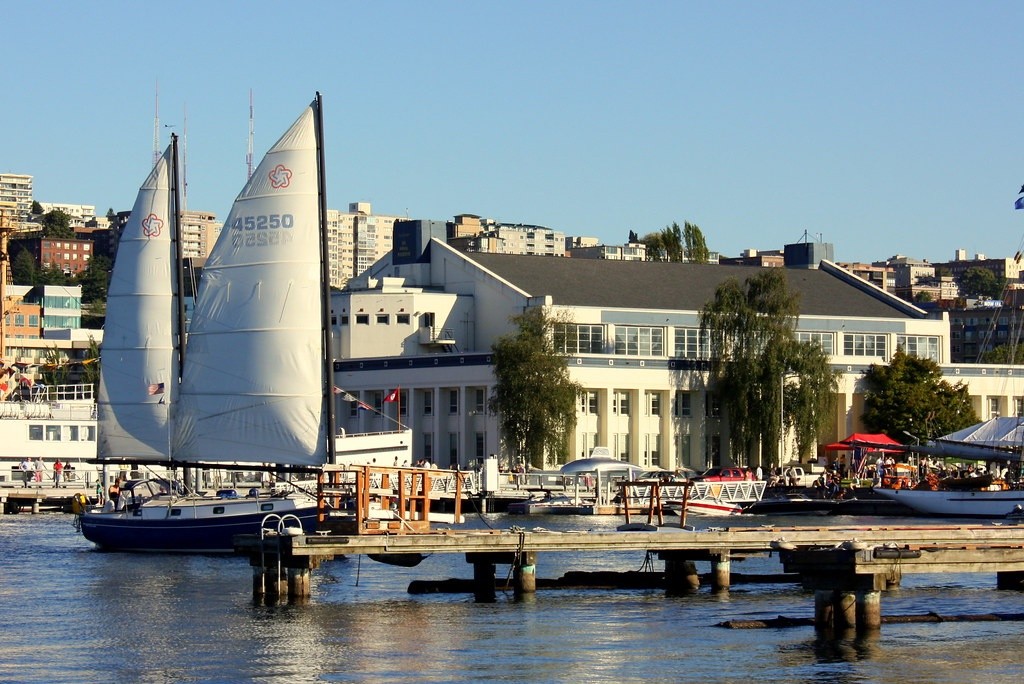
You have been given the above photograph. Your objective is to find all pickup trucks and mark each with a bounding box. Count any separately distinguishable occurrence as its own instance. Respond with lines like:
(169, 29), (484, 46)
(764, 466), (823, 488)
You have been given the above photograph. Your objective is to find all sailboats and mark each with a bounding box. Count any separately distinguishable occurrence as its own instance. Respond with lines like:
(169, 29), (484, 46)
(72, 91), (360, 556)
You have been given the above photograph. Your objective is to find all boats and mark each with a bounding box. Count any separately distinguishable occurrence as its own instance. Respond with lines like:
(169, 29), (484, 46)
(872, 488), (1024, 521)
(943, 473), (993, 491)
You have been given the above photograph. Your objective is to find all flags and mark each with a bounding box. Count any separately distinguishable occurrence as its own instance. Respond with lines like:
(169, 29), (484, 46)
(148, 383), (165, 396)
(1015, 196), (1024, 211)
(341, 393), (357, 402)
(357, 401), (372, 411)
(383, 387), (400, 403)
(333, 384), (344, 396)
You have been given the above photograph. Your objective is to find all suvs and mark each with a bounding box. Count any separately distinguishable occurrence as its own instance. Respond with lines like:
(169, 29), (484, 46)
(688, 464), (757, 485)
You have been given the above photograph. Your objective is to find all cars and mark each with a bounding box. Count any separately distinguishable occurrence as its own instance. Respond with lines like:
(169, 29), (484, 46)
(636, 470), (689, 485)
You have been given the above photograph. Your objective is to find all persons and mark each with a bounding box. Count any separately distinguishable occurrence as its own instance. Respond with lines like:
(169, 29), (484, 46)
(545, 491), (552, 500)
(367, 456), (454, 488)
(160, 484), (167, 494)
(94, 479), (105, 506)
(912, 455), (976, 479)
(611, 475), (651, 504)
(744, 464), (798, 487)
(499, 464), (527, 485)
(832, 453), (896, 481)
(21, 457), (72, 488)
(812, 470), (847, 500)
(108, 478), (126, 512)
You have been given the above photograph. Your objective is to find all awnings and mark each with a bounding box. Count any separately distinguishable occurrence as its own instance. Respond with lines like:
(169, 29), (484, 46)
(820, 432), (908, 454)
(0, 358), (98, 379)
